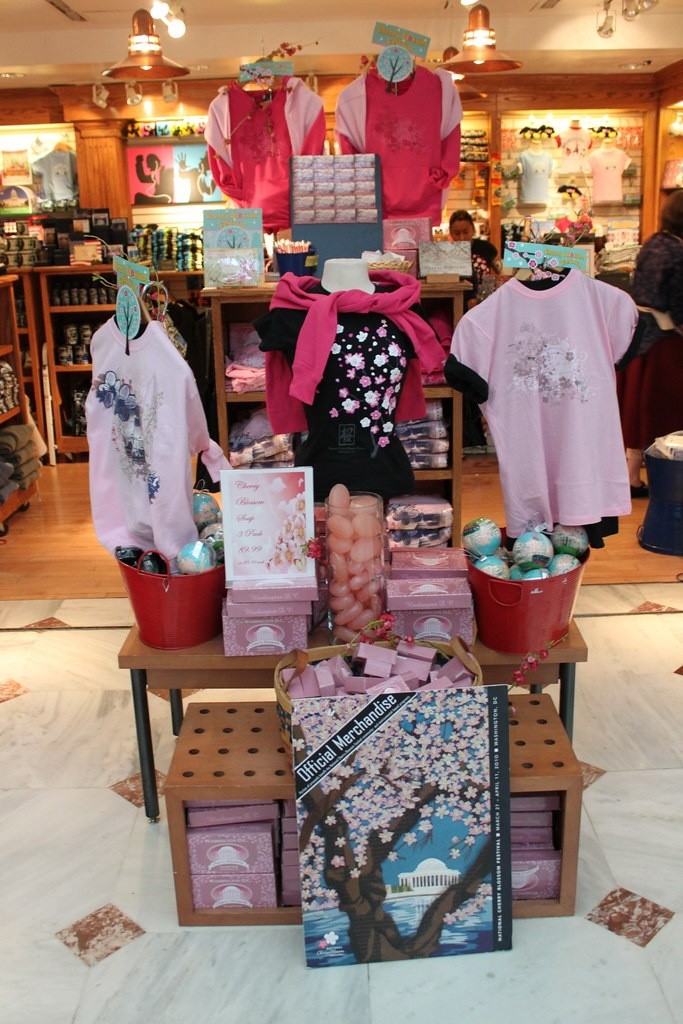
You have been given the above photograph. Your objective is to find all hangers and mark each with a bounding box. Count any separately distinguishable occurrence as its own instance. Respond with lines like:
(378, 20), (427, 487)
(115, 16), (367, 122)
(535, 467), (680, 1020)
(53, 135), (70, 152)
(234, 35), (286, 86)
(514, 265), (563, 280)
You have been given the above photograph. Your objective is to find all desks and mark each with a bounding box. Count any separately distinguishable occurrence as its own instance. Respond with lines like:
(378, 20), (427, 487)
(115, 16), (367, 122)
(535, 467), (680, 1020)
(117, 598), (589, 822)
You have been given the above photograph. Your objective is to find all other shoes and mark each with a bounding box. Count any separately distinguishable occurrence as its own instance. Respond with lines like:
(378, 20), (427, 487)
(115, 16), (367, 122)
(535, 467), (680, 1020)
(631, 481), (649, 497)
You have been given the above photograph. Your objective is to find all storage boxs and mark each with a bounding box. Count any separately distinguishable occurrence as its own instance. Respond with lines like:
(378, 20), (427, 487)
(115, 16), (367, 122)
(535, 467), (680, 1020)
(162, 547), (581, 906)
(68, 210), (130, 264)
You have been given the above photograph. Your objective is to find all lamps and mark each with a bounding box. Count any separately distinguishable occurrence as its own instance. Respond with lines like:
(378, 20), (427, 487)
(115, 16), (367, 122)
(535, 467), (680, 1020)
(92, 0), (191, 108)
(435, 0), (524, 100)
(596, 0), (659, 38)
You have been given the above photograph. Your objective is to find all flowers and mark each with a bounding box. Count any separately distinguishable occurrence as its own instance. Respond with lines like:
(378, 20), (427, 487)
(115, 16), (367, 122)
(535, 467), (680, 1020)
(543, 208), (596, 246)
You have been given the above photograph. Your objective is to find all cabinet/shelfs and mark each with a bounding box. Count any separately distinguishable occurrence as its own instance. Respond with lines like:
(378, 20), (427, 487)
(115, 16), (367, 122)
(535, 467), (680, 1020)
(0, 260), (473, 558)
(163, 694), (584, 928)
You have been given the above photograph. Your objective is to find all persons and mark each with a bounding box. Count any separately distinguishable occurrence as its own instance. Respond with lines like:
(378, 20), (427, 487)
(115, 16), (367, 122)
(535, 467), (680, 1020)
(615, 188), (683, 499)
(448, 210), (502, 284)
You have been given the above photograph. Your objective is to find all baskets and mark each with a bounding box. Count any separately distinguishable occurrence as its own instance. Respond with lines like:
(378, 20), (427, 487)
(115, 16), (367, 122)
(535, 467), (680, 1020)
(274, 640), (482, 774)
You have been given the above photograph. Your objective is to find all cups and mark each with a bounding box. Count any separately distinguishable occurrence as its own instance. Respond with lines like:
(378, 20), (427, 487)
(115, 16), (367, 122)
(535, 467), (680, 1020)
(3, 235), (39, 266)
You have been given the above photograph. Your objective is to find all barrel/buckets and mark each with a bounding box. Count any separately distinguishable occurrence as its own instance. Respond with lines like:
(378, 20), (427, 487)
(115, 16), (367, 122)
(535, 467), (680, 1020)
(465, 526), (592, 656)
(115, 550), (226, 651)
(274, 245), (316, 278)
